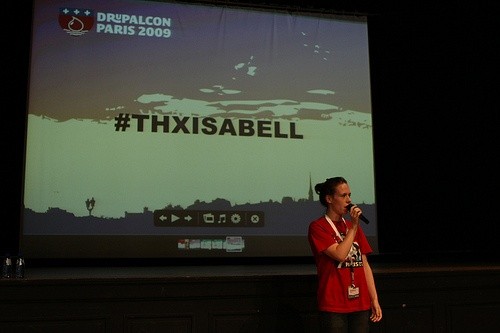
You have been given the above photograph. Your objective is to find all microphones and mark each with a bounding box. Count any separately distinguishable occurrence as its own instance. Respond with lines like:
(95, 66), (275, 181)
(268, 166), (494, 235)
(346, 204), (369, 224)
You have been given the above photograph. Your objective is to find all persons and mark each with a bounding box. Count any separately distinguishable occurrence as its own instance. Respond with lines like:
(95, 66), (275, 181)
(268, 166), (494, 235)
(308, 177), (382, 333)
